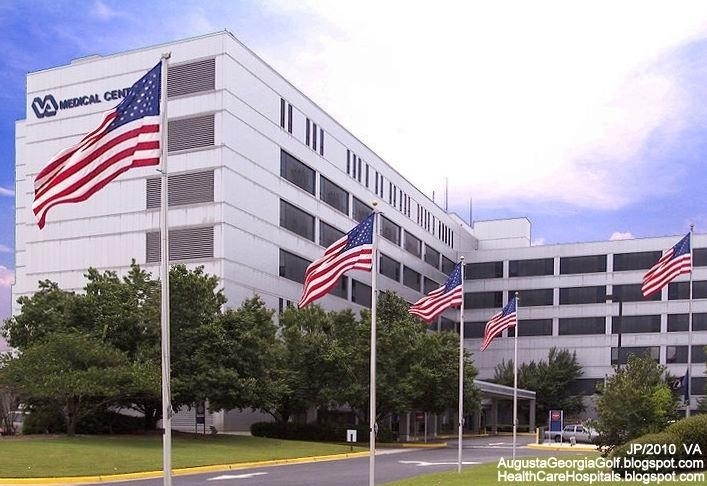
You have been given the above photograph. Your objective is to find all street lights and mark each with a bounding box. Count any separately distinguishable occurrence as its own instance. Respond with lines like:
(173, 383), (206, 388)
(605, 295), (622, 369)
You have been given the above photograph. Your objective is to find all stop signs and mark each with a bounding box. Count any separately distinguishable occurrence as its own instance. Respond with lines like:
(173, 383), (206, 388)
(552, 411), (561, 420)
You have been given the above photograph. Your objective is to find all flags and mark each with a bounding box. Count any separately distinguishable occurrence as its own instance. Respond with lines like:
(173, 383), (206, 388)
(32, 60), (163, 229)
(407, 260), (463, 323)
(298, 205), (375, 311)
(479, 294), (516, 352)
(641, 231), (693, 299)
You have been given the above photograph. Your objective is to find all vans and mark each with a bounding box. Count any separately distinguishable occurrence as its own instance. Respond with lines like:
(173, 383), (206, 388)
(2, 411), (30, 433)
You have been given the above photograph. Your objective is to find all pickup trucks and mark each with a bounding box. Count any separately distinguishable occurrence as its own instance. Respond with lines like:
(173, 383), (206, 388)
(545, 425), (599, 443)
